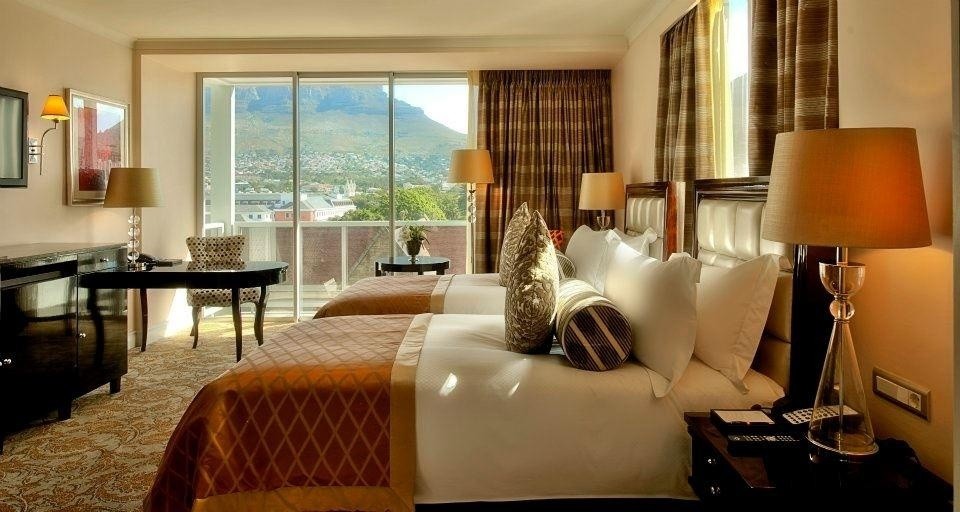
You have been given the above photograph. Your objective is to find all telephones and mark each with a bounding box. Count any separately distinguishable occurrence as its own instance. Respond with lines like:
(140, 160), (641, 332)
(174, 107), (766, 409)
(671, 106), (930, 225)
(771, 388), (862, 426)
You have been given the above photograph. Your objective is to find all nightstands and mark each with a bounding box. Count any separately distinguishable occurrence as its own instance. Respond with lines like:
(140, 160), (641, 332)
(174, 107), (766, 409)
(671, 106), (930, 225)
(684, 411), (954, 512)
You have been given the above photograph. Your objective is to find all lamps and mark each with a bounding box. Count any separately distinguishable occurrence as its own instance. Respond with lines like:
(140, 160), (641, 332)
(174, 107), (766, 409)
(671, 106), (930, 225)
(447, 149), (494, 274)
(103, 168), (167, 268)
(763, 128), (932, 464)
(579, 172), (625, 231)
(28, 95), (70, 175)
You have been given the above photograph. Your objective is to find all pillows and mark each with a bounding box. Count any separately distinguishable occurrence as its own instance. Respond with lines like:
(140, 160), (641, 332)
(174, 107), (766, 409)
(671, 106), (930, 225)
(564, 225), (657, 288)
(553, 278), (633, 373)
(555, 249), (575, 284)
(668, 252), (791, 395)
(499, 202), (531, 287)
(549, 230), (564, 253)
(594, 229), (701, 398)
(505, 211), (560, 355)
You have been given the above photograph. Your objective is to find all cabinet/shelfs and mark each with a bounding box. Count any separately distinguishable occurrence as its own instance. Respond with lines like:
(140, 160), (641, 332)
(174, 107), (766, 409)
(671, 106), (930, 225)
(0, 242), (127, 455)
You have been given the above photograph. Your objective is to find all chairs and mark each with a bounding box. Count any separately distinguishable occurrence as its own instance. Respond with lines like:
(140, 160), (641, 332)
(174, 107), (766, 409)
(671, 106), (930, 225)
(186, 234), (269, 350)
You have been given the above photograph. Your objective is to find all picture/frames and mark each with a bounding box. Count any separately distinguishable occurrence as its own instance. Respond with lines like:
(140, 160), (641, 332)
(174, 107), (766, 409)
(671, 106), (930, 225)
(66, 88), (132, 208)
(0, 86), (28, 188)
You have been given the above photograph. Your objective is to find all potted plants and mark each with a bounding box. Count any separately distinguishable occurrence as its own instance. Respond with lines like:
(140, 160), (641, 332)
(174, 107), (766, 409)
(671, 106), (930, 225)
(403, 223), (430, 262)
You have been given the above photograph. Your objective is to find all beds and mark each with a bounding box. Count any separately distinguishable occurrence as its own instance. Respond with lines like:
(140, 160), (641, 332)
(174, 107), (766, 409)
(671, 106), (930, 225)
(143, 176), (837, 512)
(312, 181), (684, 319)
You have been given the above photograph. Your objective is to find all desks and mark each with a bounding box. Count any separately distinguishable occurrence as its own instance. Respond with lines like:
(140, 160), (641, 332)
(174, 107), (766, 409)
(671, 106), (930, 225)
(80, 261), (289, 363)
(375, 256), (449, 277)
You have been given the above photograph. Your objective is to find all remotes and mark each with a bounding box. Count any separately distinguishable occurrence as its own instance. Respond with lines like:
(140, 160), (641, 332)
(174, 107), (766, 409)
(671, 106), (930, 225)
(726, 434), (803, 447)
